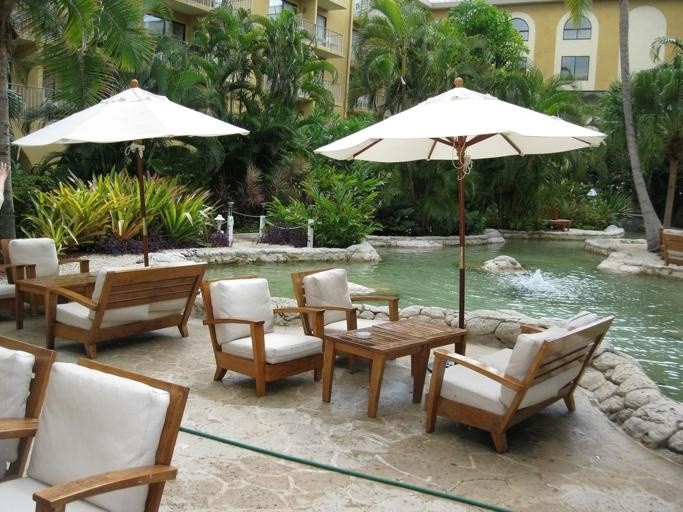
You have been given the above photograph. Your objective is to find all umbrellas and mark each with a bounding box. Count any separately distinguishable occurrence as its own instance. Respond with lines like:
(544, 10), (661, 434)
(312, 77), (611, 327)
(8, 78), (250, 265)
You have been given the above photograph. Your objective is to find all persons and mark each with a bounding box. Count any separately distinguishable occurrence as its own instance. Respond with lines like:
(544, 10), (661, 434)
(0, 161), (11, 212)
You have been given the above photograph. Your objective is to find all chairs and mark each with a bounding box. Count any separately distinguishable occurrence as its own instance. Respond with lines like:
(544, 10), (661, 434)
(0, 237), (89, 317)
(200, 266), (401, 398)
(662, 228), (682, 266)
(0, 334), (190, 511)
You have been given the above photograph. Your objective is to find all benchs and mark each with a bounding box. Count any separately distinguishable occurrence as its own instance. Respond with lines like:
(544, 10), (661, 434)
(43, 261), (209, 360)
(421, 311), (615, 453)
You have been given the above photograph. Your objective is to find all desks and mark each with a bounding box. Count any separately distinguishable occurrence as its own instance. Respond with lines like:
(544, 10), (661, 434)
(321, 319), (469, 418)
(12, 272), (100, 329)
